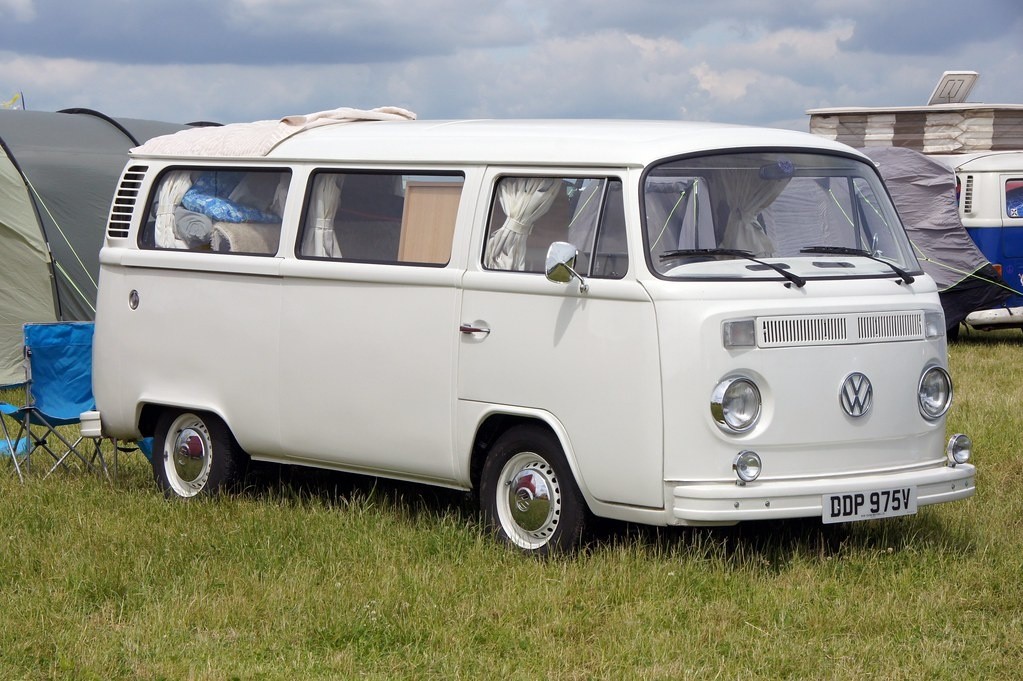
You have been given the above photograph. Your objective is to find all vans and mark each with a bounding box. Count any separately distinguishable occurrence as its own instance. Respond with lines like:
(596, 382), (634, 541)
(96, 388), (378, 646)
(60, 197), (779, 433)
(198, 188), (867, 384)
(92, 117), (977, 563)
(927, 150), (1023, 342)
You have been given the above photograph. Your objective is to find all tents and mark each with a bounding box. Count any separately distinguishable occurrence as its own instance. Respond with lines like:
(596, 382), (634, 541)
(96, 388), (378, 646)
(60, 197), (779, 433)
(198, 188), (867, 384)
(1, 103), (224, 388)
(571, 147), (1014, 329)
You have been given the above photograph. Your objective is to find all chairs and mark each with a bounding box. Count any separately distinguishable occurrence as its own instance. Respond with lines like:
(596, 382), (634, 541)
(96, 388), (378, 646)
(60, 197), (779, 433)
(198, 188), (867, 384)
(0, 321), (154, 485)
